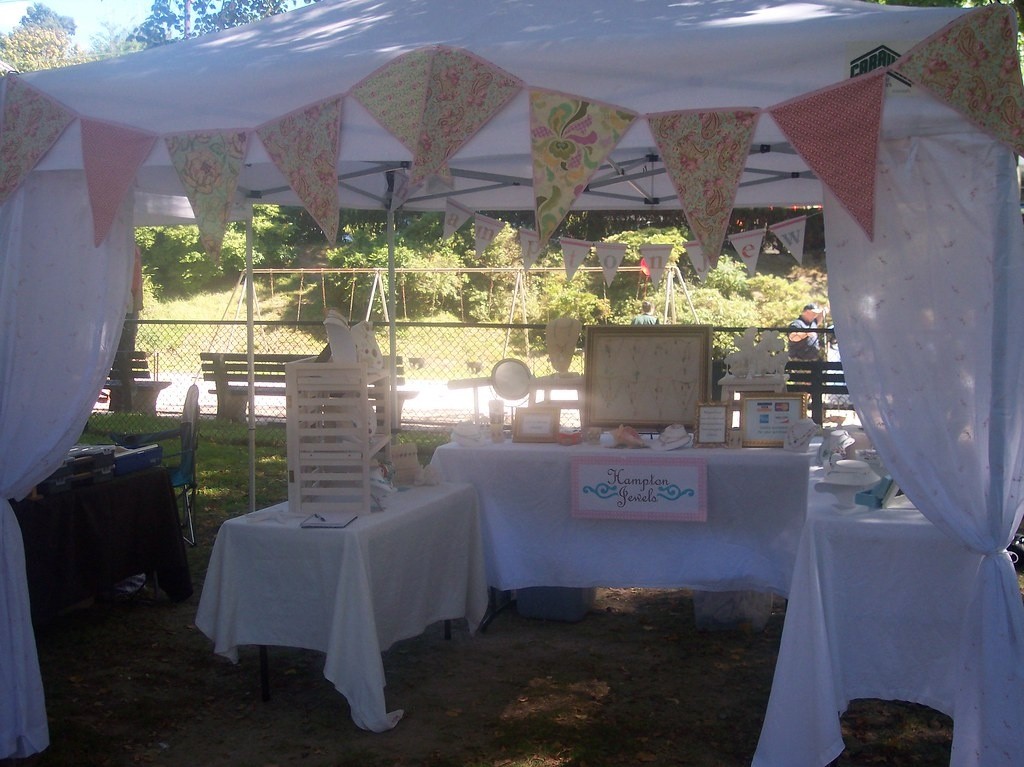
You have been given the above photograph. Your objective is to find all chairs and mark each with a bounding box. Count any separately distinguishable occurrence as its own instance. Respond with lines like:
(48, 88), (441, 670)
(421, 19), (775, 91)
(108, 383), (200, 546)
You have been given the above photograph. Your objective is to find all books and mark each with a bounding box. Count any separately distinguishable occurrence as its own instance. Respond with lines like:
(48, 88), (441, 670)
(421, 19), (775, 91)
(300, 511), (360, 528)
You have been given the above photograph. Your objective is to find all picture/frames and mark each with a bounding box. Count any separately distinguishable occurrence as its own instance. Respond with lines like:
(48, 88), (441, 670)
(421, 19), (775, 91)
(513, 406), (562, 443)
(693, 402), (733, 449)
(740, 391), (808, 448)
(583, 324), (713, 427)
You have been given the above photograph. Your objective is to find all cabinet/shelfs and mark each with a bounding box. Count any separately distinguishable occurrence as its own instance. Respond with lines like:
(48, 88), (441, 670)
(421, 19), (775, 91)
(285, 356), (391, 512)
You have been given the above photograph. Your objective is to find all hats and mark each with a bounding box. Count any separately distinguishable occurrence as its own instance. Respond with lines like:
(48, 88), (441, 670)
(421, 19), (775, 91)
(803, 304), (822, 314)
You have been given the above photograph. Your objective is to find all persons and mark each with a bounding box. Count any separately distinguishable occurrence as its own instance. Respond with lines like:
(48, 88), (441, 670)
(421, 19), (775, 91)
(630, 303), (661, 325)
(788, 303), (830, 386)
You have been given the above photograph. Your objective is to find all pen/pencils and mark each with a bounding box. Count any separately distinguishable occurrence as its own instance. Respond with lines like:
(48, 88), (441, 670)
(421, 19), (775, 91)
(314, 513), (326, 522)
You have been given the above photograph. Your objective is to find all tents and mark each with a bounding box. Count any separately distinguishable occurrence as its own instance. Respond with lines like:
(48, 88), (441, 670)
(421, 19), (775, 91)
(0, 0), (1024, 767)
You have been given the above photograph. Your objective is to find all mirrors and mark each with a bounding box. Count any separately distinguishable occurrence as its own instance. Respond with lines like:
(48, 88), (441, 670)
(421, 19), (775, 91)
(491, 359), (532, 400)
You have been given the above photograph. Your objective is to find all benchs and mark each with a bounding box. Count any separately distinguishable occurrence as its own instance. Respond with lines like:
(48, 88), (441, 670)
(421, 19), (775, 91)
(102, 349), (172, 414)
(200, 352), (419, 431)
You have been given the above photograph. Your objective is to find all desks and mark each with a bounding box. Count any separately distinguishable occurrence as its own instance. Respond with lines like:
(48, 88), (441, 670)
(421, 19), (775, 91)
(435, 433), (815, 635)
(751, 429), (1024, 767)
(6, 467), (192, 627)
(194, 482), (488, 733)
